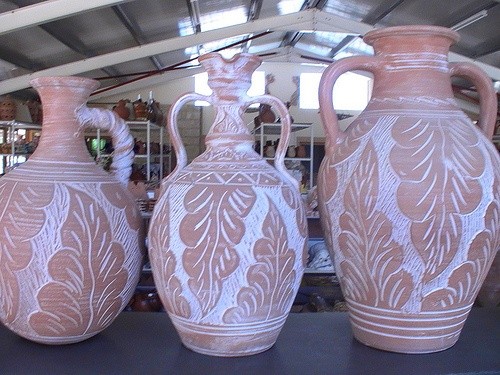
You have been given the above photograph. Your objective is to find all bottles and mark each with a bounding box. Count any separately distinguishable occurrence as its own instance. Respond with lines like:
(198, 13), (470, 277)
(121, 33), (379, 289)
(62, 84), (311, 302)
(317, 25), (500, 354)
(0, 77), (145, 345)
(148, 53), (308, 357)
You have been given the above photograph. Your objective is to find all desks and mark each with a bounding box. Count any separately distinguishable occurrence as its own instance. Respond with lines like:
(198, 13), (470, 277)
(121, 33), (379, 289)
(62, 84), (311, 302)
(0, 313), (500, 375)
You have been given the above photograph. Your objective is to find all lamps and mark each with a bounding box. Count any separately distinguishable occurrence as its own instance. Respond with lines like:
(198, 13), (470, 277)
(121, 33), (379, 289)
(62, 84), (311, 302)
(450, 9), (487, 32)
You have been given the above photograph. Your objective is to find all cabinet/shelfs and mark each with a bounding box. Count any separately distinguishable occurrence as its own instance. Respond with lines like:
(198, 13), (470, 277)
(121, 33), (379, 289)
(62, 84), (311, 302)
(251, 121), (313, 194)
(0, 120), (42, 176)
(97, 120), (172, 289)
